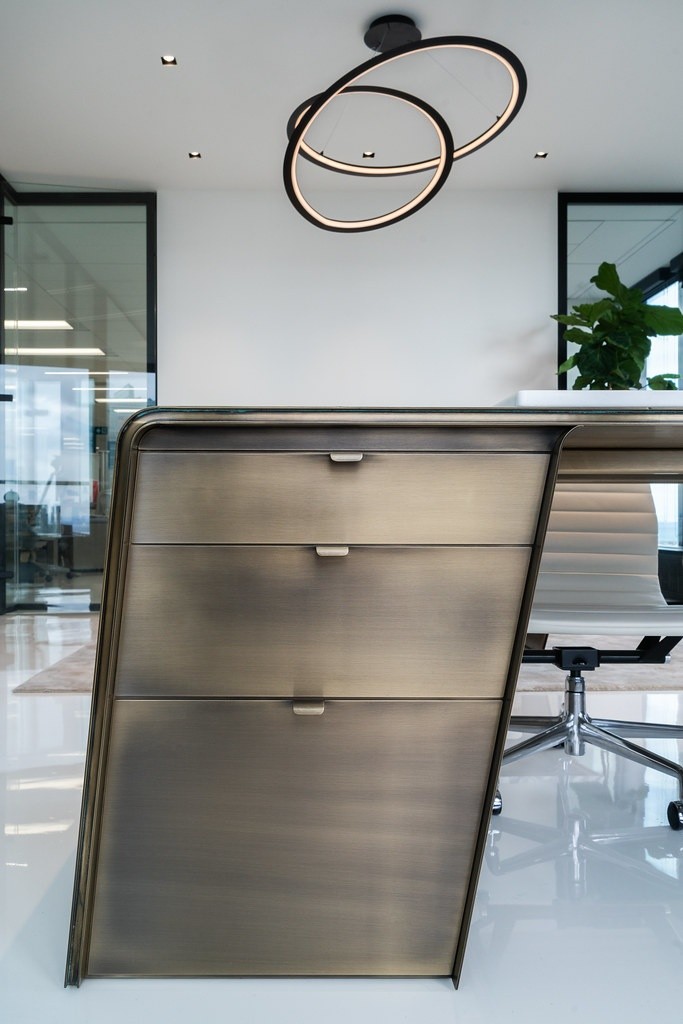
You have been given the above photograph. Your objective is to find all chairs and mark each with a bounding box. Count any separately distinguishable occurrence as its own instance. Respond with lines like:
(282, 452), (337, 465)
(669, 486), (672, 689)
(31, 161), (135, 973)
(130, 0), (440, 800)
(493, 484), (683, 830)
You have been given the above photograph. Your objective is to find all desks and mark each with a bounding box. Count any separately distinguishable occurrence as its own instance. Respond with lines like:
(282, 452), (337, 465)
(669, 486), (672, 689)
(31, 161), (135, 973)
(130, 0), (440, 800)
(64, 405), (683, 988)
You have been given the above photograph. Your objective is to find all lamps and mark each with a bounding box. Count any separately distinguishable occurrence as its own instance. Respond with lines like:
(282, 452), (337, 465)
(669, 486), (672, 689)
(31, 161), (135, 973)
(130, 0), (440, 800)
(283, 14), (528, 234)
